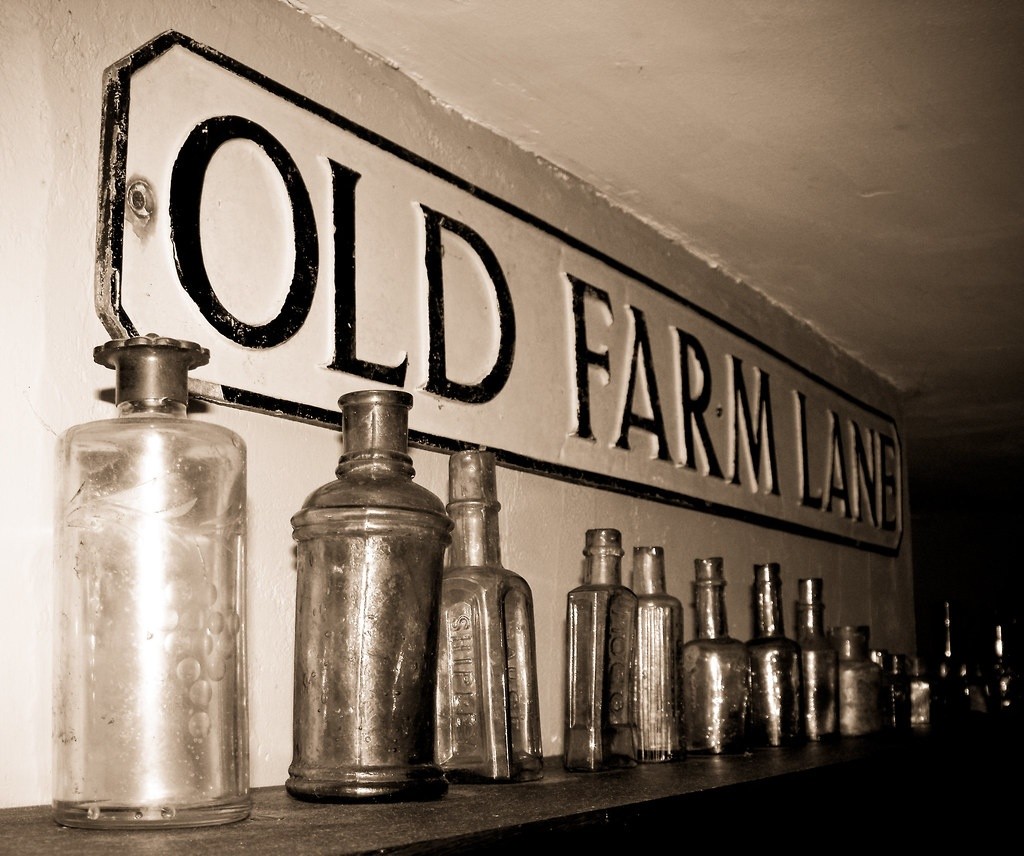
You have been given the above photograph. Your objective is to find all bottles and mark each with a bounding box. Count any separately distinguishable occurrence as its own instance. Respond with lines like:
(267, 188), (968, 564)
(285, 392), (454, 803)
(433, 451), (543, 784)
(563, 527), (1024, 775)
(54, 336), (251, 829)
